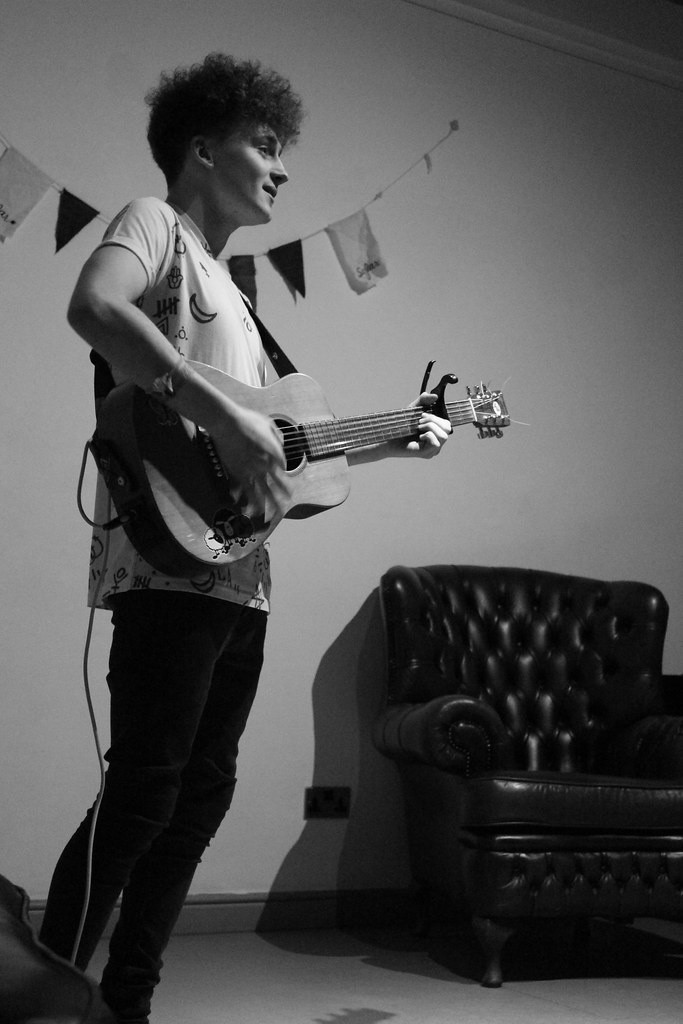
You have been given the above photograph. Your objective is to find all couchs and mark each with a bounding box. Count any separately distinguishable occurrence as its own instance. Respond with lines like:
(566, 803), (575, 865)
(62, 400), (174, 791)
(370, 563), (683, 987)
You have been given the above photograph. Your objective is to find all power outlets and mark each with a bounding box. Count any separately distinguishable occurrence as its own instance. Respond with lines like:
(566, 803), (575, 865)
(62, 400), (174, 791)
(303, 786), (352, 821)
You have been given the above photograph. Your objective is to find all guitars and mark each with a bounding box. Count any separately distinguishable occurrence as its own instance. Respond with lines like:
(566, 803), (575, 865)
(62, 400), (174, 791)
(89, 355), (511, 579)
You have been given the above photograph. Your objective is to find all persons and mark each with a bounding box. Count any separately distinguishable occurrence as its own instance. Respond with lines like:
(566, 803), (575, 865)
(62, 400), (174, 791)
(39, 51), (453, 1024)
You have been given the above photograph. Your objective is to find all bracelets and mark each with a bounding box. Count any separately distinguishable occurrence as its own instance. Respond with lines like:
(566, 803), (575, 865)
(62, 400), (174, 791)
(149, 357), (195, 406)
